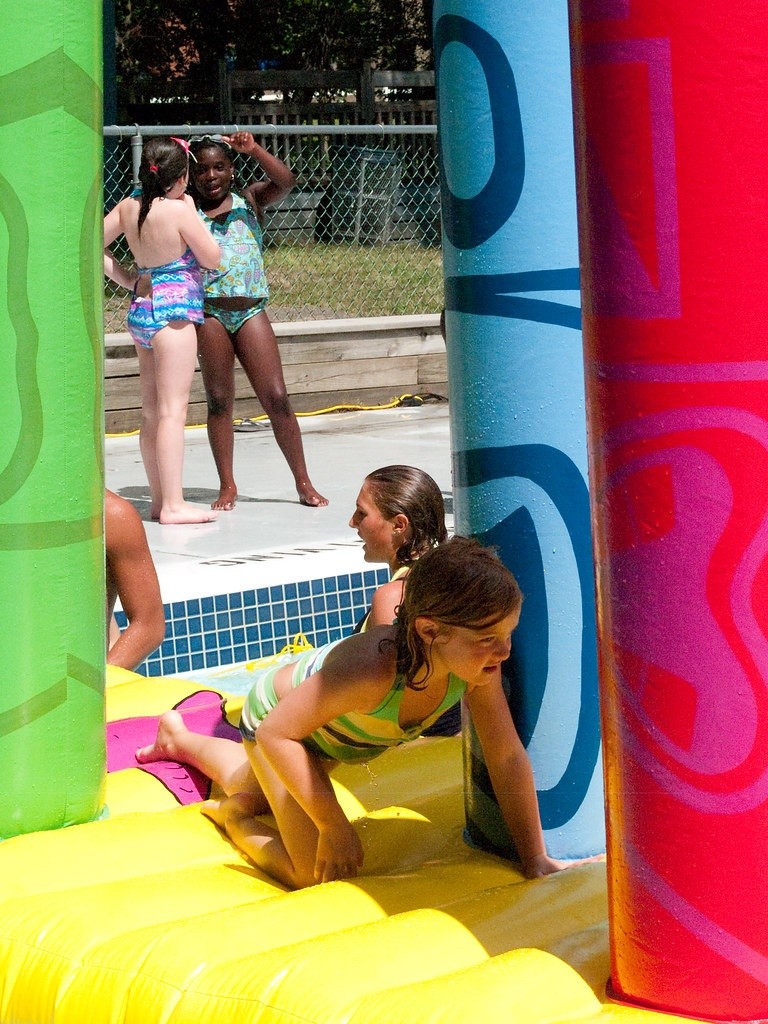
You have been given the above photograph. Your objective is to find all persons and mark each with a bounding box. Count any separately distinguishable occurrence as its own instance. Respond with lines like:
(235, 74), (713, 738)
(134, 534), (563, 893)
(189, 131), (331, 511)
(105, 489), (165, 672)
(348, 463), (460, 736)
(102, 137), (225, 526)
